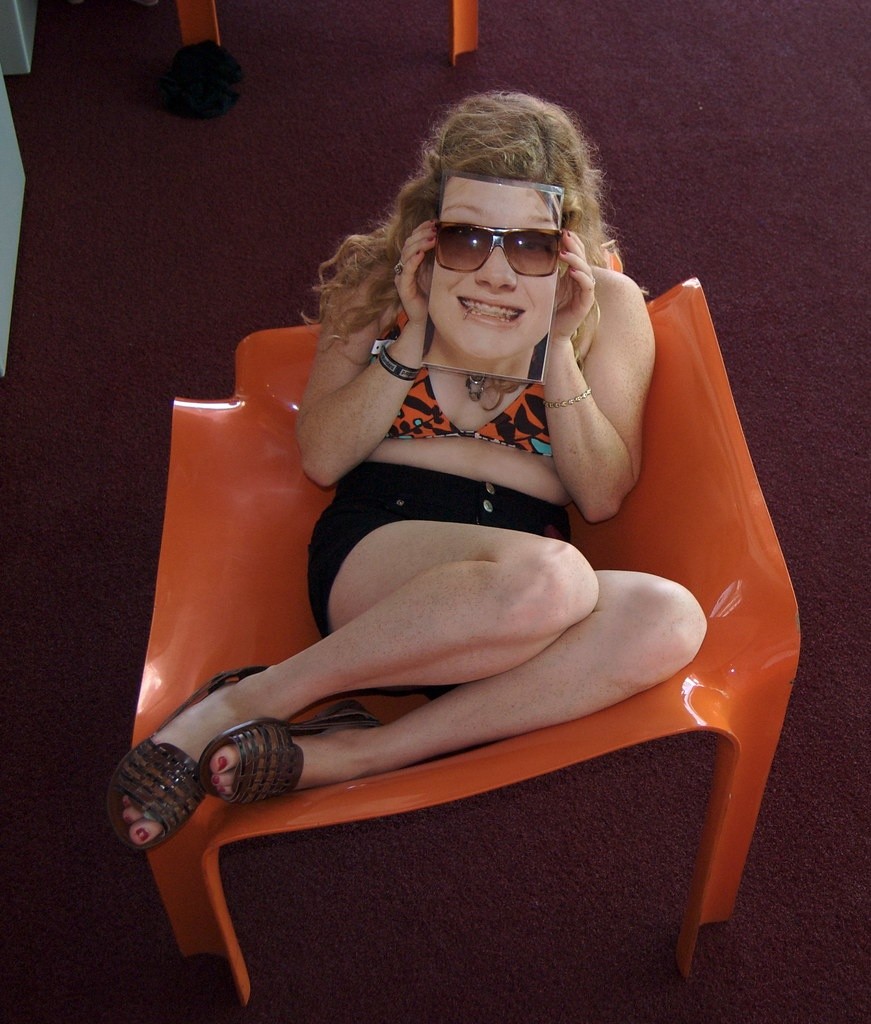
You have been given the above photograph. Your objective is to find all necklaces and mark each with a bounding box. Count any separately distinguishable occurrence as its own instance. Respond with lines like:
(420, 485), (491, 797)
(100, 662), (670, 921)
(465, 374), (487, 400)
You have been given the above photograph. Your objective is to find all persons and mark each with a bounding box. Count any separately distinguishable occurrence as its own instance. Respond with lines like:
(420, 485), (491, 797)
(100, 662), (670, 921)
(422, 174), (564, 383)
(108, 79), (710, 851)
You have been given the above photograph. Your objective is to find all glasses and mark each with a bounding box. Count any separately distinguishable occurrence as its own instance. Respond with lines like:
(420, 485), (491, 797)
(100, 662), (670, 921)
(436, 221), (563, 278)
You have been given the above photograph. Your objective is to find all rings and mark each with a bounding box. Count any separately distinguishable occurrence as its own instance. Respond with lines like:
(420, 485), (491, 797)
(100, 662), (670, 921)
(393, 260), (405, 275)
(588, 276), (596, 291)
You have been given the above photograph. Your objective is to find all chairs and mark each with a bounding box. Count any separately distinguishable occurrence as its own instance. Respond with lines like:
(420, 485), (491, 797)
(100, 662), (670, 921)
(129, 277), (801, 1008)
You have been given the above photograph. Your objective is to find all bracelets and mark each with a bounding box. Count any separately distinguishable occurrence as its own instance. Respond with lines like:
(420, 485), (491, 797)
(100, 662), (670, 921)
(370, 338), (422, 381)
(542, 386), (592, 408)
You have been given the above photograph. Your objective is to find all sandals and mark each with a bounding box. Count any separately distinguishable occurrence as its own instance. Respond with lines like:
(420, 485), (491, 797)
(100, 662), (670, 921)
(105, 668), (274, 852)
(197, 698), (381, 806)
